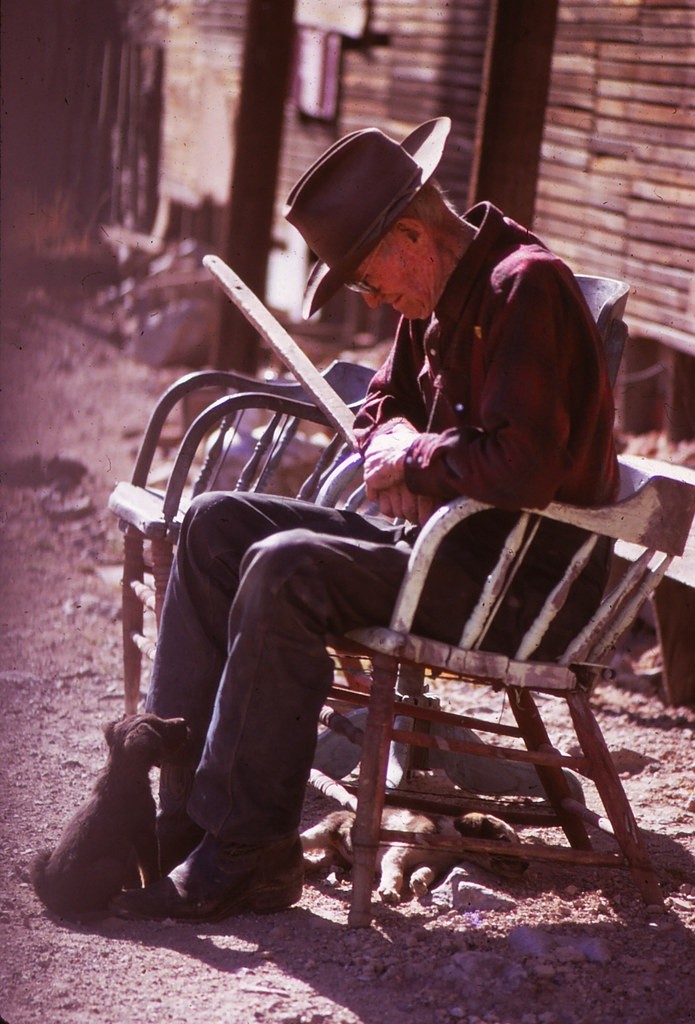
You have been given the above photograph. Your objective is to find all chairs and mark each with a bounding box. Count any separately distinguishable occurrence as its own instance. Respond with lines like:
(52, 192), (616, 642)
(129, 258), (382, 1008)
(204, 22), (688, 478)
(107, 360), (695, 933)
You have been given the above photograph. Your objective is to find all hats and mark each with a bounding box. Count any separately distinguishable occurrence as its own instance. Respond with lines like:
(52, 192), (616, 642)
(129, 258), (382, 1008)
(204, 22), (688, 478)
(287, 115), (452, 319)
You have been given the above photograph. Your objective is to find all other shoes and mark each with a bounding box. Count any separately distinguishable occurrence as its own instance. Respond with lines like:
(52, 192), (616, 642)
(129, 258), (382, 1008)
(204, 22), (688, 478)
(126, 802), (208, 886)
(110, 834), (305, 920)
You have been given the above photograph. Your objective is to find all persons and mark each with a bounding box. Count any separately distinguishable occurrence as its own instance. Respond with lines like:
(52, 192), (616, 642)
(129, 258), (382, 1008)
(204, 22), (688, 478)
(108, 115), (621, 925)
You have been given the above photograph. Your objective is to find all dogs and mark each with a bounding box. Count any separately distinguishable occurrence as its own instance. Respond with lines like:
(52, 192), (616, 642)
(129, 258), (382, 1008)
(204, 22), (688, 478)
(297, 810), (530, 903)
(28, 713), (190, 926)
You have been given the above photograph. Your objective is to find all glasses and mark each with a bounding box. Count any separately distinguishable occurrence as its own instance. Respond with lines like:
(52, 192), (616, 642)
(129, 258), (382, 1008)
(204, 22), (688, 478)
(342, 230), (392, 293)
